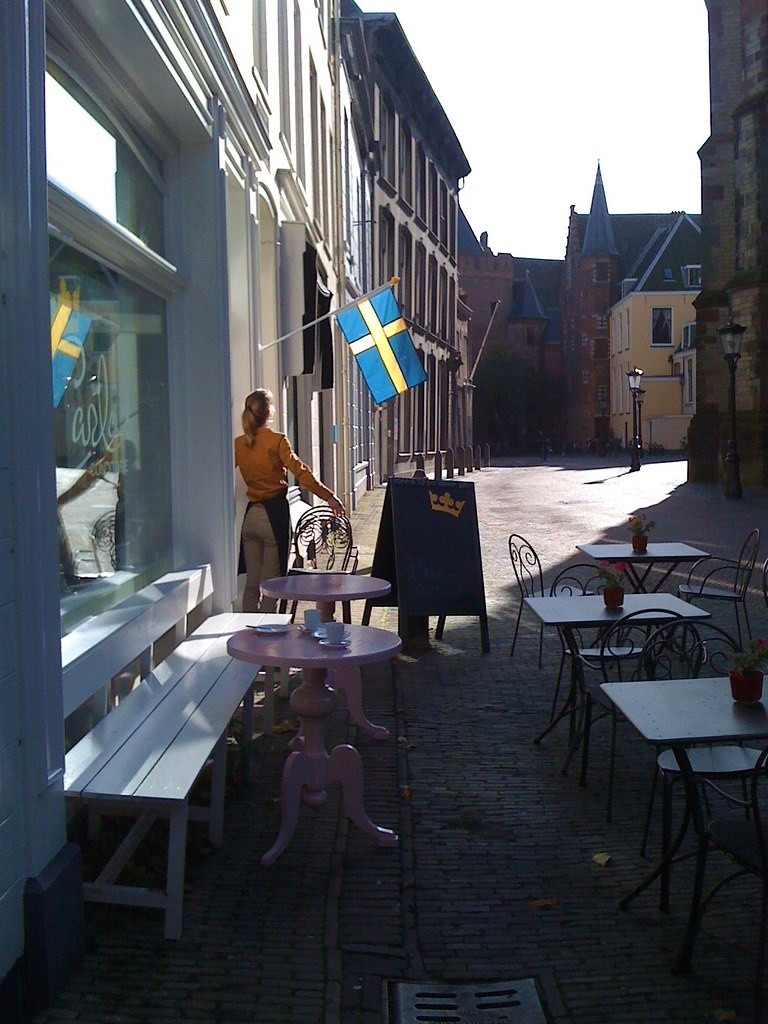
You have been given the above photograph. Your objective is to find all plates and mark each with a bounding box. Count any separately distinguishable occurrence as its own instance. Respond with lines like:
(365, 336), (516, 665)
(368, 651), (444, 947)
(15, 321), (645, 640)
(253, 624), (295, 632)
(298, 625), (352, 647)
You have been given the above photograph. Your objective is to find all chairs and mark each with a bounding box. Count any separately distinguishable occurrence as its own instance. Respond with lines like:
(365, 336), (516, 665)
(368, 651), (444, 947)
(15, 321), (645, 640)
(547, 558), (768, 932)
(678, 528), (760, 659)
(511, 535), (595, 669)
(278, 506), (359, 625)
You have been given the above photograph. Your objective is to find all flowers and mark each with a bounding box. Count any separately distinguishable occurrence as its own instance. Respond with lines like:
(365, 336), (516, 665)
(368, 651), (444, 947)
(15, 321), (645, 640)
(593, 560), (630, 587)
(729, 639), (768, 674)
(626, 513), (656, 535)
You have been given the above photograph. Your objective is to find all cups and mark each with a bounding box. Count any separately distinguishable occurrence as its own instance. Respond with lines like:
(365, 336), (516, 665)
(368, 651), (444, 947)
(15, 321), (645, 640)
(304, 609), (320, 630)
(326, 622), (344, 645)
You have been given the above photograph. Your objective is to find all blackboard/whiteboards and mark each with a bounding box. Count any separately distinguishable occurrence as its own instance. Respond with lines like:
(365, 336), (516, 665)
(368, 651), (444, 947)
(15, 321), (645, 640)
(362, 476), (490, 617)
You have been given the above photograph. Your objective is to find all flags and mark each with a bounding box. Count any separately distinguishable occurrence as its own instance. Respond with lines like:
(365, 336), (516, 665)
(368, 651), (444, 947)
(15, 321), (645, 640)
(50, 295), (94, 409)
(333, 287), (430, 405)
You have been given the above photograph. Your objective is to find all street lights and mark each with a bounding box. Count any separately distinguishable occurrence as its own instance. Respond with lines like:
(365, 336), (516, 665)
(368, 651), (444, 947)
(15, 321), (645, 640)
(625, 366), (647, 471)
(717, 314), (749, 499)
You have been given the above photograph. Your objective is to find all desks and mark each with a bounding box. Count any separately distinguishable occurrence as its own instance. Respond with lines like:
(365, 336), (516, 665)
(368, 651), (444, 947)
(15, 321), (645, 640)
(522, 593), (711, 775)
(599, 673), (768, 978)
(227, 621), (402, 864)
(576, 543), (712, 670)
(259, 573), (390, 739)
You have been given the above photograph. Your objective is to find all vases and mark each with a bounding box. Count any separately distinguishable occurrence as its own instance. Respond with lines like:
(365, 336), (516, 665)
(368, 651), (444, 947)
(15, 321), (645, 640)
(604, 586), (625, 607)
(728, 671), (764, 702)
(631, 535), (648, 553)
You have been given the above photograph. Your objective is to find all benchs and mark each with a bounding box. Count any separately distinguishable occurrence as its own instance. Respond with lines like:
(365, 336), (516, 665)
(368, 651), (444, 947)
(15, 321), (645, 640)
(61, 563), (292, 941)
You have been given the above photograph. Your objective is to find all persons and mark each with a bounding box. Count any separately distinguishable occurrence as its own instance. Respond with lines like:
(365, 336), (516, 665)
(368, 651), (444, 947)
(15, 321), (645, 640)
(236, 391), (345, 612)
(56, 432), (125, 571)
